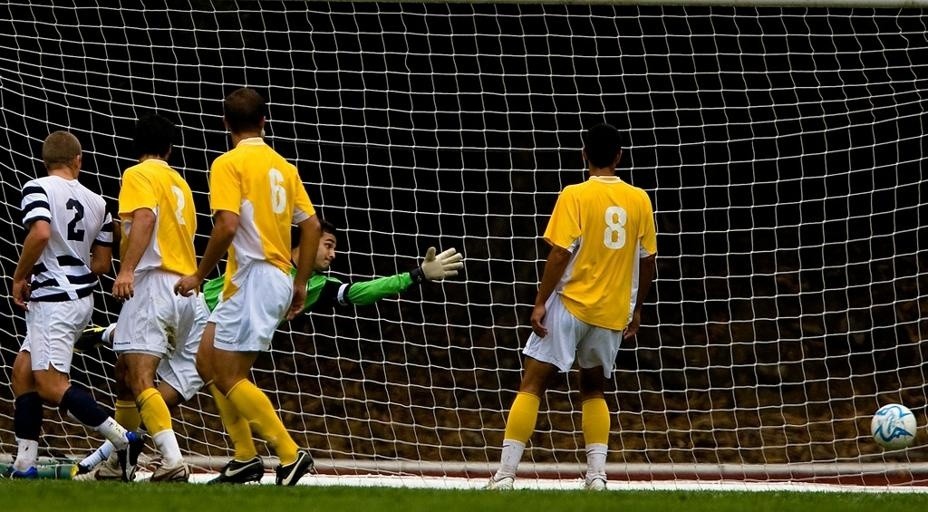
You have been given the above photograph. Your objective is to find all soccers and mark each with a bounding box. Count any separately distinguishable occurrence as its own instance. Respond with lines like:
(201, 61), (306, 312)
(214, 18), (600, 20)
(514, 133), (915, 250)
(869, 405), (916, 451)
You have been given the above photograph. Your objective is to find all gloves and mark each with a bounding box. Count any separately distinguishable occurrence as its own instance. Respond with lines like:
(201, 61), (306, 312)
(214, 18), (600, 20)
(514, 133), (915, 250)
(421, 245), (465, 280)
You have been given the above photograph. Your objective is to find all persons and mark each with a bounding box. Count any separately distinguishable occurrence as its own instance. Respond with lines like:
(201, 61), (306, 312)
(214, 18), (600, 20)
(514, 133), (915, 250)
(172, 90), (323, 487)
(111, 113), (198, 482)
(72, 219), (464, 476)
(481, 123), (658, 492)
(0, 130), (144, 483)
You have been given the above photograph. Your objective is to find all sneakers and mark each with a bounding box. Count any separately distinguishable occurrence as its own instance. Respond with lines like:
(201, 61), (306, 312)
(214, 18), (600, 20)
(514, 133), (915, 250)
(73, 326), (107, 352)
(0, 464), (40, 480)
(205, 454), (265, 487)
(478, 474), (515, 492)
(584, 470), (608, 493)
(70, 462), (91, 479)
(69, 460), (123, 482)
(274, 448), (314, 488)
(116, 430), (148, 483)
(136, 459), (192, 483)
